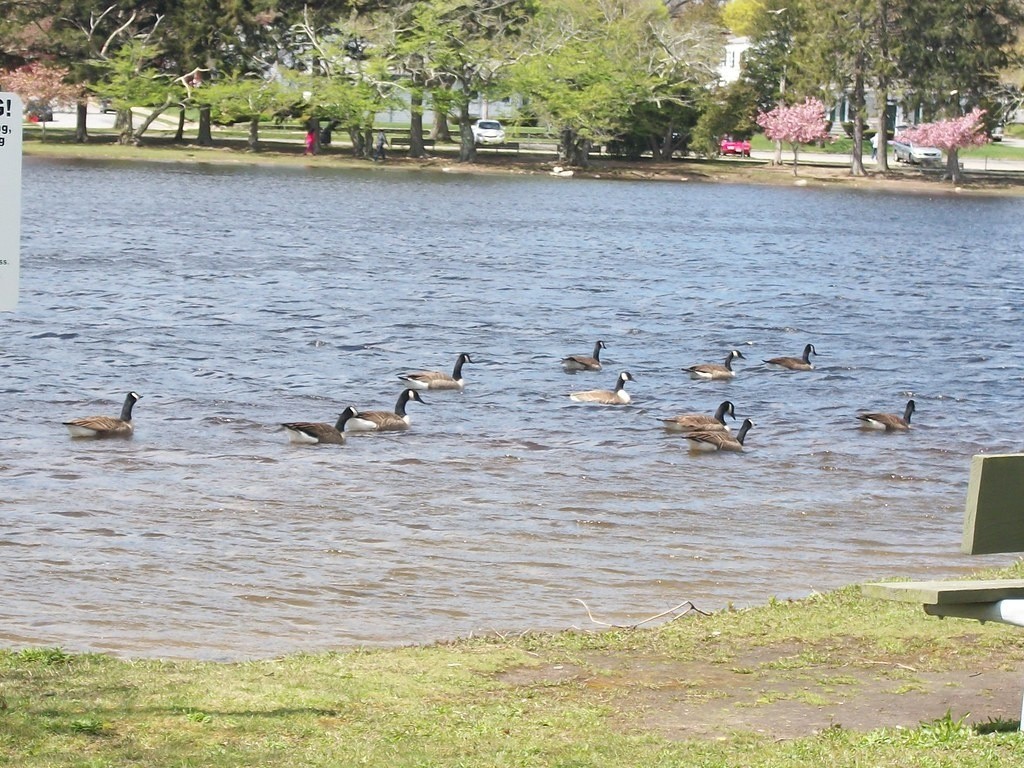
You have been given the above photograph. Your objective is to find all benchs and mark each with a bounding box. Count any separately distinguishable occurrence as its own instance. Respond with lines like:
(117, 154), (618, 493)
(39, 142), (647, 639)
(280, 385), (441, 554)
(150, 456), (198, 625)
(917, 160), (965, 175)
(391, 137), (435, 150)
(860, 452), (1024, 733)
(474, 140), (520, 155)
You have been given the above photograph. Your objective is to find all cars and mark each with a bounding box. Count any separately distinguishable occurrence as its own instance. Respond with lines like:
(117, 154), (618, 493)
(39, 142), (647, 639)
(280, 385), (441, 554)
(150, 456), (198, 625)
(24, 100), (53, 120)
(893, 137), (943, 165)
(99, 98), (115, 112)
(720, 134), (752, 157)
(469, 119), (504, 146)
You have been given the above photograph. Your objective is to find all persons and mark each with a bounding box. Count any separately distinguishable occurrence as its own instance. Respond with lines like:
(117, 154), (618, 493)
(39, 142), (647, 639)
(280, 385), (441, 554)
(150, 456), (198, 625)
(370, 128), (389, 162)
(870, 132), (880, 160)
(304, 127), (316, 157)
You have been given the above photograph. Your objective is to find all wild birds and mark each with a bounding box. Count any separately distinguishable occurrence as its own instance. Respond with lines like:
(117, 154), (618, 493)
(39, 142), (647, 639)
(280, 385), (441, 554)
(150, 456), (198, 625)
(761, 343), (818, 371)
(560, 371), (637, 405)
(855, 400), (917, 432)
(280, 405), (364, 445)
(61, 391), (145, 439)
(396, 352), (475, 392)
(334, 389), (427, 431)
(682, 349), (746, 380)
(559, 340), (607, 371)
(656, 400), (737, 432)
(681, 418), (758, 454)
